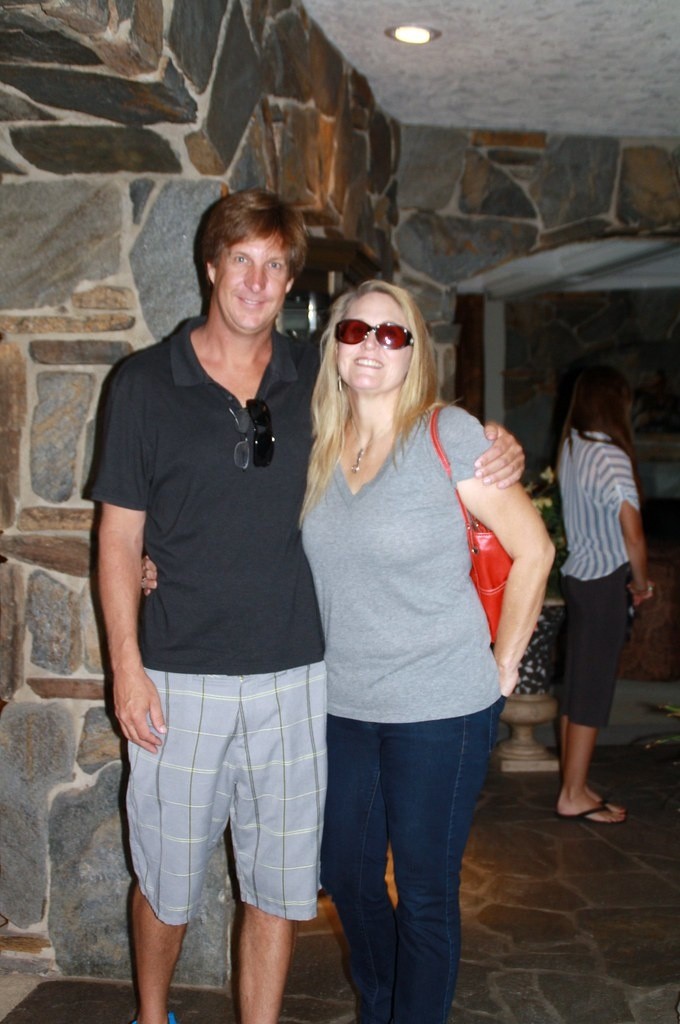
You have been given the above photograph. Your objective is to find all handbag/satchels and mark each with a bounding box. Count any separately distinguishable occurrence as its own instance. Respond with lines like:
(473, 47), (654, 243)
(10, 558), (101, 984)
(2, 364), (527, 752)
(429, 404), (540, 648)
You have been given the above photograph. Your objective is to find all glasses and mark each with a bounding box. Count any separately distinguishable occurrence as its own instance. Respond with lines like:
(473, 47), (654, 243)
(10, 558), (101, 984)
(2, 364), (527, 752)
(228, 407), (250, 473)
(246, 398), (275, 468)
(334, 319), (414, 351)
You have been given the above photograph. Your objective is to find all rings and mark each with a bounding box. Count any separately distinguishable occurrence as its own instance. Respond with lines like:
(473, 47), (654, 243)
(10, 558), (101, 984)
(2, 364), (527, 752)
(142, 578), (147, 589)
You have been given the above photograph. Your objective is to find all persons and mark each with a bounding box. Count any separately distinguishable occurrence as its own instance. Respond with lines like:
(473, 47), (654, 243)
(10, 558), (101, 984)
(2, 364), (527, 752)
(555, 360), (649, 824)
(140, 280), (557, 1023)
(80, 189), (528, 1023)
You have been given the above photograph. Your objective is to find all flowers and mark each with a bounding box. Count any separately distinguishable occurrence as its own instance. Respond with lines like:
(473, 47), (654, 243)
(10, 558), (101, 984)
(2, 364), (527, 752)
(523, 466), (567, 599)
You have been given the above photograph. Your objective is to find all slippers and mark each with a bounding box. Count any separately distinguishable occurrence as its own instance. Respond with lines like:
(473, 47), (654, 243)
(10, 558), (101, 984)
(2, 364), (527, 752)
(555, 789), (627, 824)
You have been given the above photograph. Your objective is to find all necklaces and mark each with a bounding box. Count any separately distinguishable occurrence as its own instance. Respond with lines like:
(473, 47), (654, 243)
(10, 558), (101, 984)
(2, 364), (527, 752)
(349, 413), (391, 473)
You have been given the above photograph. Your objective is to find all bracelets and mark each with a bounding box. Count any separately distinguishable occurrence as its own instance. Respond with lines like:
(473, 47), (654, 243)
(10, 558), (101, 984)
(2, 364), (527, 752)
(626, 579), (657, 601)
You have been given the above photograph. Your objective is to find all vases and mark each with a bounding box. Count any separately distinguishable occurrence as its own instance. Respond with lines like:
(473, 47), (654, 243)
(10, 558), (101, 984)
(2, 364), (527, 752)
(490, 599), (566, 772)
